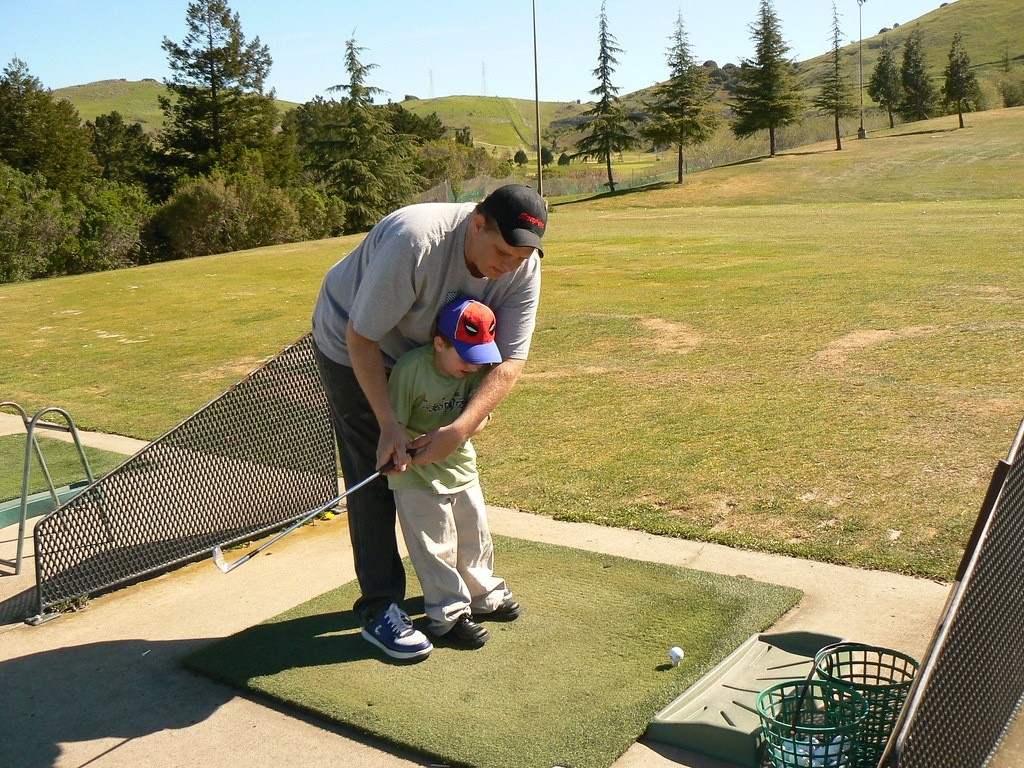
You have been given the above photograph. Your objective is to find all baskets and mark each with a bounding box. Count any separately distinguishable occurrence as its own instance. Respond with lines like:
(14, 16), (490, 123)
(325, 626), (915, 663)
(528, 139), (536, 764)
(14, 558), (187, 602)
(755, 653), (870, 768)
(814, 642), (920, 768)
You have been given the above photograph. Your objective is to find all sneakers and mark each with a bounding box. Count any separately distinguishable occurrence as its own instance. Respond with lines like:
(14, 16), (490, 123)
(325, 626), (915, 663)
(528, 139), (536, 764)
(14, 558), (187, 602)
(361, 602), (433, 659)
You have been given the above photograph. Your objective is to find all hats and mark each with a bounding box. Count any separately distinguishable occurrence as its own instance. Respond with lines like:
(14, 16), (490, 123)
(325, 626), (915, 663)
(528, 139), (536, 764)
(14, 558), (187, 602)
(438, 299), (503, 364)
(494, 184), (548, 259)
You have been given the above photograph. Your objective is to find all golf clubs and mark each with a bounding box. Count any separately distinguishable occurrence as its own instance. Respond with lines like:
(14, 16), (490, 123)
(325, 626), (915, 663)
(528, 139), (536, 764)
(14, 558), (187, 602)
(212, 447), (419, 576)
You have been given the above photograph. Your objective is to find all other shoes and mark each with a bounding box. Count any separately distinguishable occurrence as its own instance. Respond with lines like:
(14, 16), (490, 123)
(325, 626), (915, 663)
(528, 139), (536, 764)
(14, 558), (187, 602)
(490, 599), (520, 619)
(440, 613), (491, 644)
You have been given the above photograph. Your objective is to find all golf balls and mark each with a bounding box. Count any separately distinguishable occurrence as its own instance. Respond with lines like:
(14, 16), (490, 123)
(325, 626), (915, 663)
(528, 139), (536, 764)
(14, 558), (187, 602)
(669, 646), (684, 661)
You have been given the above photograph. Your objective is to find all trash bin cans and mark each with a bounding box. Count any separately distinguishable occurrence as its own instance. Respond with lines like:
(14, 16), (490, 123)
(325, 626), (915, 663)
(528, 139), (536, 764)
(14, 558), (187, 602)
(814, 640), (921, 767)
(755, 680), (869, 768)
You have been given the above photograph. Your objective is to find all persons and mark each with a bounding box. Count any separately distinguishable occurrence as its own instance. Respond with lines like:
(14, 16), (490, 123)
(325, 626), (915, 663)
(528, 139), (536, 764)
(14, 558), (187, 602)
(388, 298), (521, 650)
(311, 184), (548, 662)
